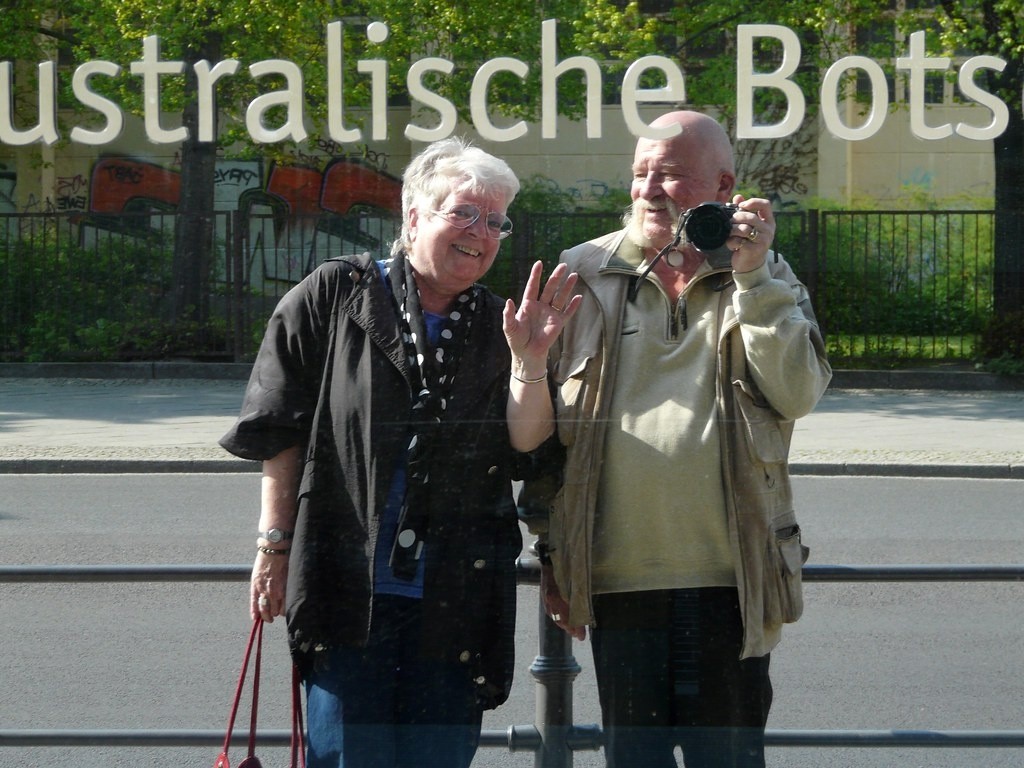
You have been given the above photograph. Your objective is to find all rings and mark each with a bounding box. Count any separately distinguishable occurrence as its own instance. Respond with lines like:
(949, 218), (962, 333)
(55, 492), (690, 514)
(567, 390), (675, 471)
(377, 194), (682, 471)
(747, 229), (758, 240)
(550, 305), (561, 312)
(738, 239), (746, 248)
(258, 598), (270, 606)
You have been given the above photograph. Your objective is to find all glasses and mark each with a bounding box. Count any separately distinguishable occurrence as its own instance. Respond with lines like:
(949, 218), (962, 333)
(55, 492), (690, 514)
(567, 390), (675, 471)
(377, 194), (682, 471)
(424, 203), (513, 240)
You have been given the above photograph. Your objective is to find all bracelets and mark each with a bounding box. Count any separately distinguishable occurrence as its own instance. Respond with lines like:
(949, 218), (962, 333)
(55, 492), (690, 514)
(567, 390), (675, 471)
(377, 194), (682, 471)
(257, 546), (291, 555)
(510, 368), (548, 384)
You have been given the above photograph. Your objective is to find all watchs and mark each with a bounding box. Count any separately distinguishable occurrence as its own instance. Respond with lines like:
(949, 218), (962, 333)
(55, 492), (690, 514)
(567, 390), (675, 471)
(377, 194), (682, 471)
(257, 528), (293, 543)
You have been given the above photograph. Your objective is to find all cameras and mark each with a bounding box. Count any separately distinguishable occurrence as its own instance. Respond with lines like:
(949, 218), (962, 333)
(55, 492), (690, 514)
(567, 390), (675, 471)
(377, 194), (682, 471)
(684, 201), (748, 268)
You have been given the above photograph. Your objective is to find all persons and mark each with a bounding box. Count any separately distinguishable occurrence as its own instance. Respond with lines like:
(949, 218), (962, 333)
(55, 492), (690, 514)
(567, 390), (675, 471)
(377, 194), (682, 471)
(517, 110), (834, 767)
(217, 135), (583, 768)
